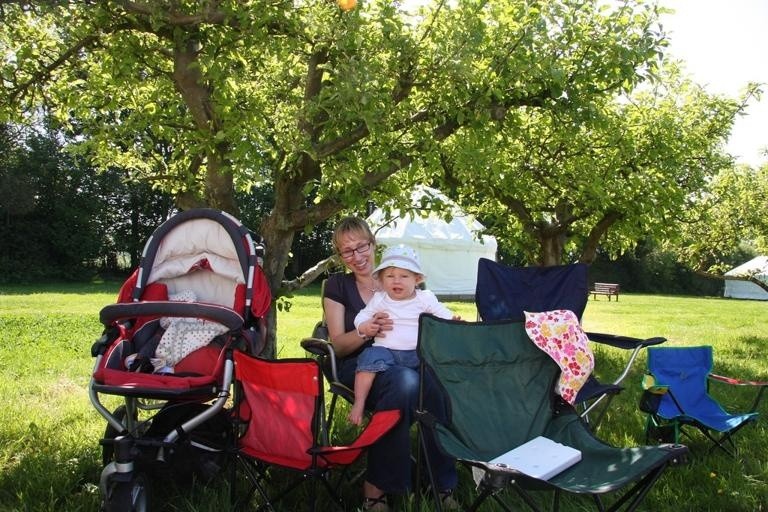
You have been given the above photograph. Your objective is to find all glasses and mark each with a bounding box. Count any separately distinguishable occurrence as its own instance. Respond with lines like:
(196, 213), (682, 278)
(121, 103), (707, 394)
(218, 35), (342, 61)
(337, 240), (372, 259)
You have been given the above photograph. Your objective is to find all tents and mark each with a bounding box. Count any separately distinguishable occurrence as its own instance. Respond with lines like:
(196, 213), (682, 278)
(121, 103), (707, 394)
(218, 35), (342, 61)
(724, 256), (768, 301)
(365, 184), (497, 295)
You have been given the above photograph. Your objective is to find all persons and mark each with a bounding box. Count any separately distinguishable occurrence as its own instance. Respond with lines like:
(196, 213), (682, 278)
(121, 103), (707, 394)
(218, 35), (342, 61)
(348, 242), (466, 426)
(321, 217), (463, 512)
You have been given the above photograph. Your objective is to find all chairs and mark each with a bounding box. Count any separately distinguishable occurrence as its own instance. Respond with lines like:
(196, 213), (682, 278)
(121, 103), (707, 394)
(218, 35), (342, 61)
(474, 255), (666, 437)
(591, 281), (620, 302)
(644, 343), (759, 457)
(418, 310), (686, 512)
(301, 279), (430, 475)
(225, 347), (401, 512)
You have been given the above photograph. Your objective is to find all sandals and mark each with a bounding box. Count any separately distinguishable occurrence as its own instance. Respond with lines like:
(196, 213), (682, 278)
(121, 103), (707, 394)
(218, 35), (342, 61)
(362, 478), (387, 512)
(438, 488), (459, 512)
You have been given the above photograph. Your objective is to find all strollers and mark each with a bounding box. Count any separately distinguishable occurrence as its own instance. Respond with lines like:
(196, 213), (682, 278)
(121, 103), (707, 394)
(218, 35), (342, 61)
(89, 208), (273, 512)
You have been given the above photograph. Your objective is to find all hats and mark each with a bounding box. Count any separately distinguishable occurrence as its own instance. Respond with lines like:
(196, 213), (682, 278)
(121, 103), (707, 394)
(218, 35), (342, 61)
(370, 247), (426, 280)
